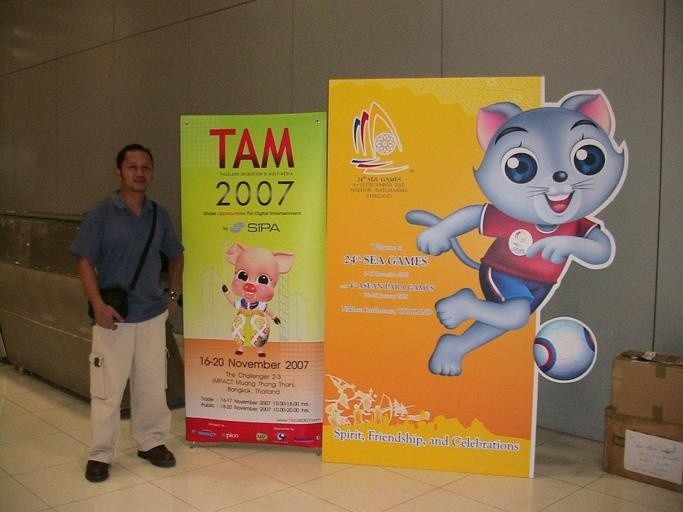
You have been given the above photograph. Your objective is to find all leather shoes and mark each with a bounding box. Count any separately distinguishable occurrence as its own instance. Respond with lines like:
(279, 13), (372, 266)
(136, 444), (176, 468)
(84, 459), (109, 482)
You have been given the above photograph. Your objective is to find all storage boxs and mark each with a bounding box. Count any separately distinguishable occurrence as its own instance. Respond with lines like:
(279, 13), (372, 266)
(601, 349), (682, 494)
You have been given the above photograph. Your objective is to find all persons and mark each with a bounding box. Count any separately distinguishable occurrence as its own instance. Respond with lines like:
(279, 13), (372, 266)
(73, 143), (185, 481)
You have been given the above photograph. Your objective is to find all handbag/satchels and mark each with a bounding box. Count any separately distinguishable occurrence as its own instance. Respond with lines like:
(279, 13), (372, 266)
(87, 284), (128, 320)
